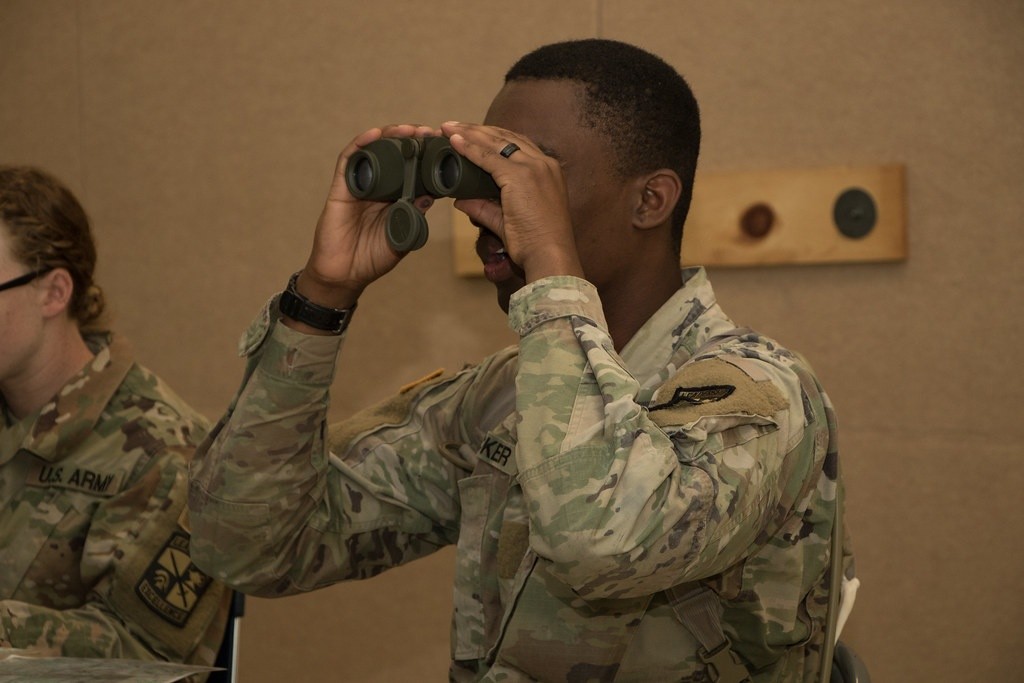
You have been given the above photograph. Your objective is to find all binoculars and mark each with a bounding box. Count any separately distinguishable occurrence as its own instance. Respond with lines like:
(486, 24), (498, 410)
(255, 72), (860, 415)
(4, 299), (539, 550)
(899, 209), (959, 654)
(345, 136), (501, 201)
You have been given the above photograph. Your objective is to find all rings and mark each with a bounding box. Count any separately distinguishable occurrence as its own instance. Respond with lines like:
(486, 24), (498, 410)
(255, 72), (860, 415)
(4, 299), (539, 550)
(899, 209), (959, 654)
(500, 143), (520, 158)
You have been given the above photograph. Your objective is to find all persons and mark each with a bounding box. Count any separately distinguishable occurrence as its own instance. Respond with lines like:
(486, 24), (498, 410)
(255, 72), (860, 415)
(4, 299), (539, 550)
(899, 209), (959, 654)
(0, 164), (233, 683)
(188, 39), (857, 683)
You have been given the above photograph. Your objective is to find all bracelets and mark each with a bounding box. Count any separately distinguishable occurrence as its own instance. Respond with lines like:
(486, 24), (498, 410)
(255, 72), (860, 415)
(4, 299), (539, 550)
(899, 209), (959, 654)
(279, 271), (357, 336)
